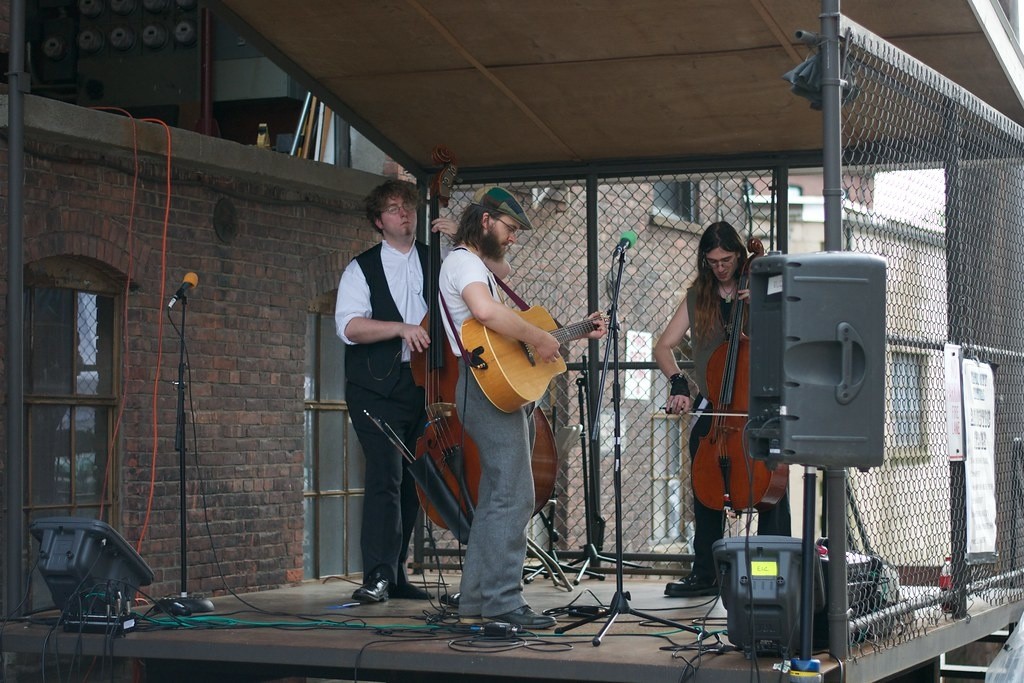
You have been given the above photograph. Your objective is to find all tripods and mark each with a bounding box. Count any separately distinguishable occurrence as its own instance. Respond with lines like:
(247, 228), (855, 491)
(523, 253), (710, 646)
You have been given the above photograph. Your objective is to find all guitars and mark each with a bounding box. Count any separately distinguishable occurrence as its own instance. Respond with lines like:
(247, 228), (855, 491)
(460, 305), (621, 415)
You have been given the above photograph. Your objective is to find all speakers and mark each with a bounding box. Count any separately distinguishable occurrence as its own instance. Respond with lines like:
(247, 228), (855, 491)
(30, 516), (155, 617)
(712, 535), (825, 657)
(747, 251), (887, 472)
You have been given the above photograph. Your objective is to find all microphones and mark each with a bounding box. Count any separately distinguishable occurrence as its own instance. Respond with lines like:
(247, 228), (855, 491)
(168, 272), (198, 309)
(613, 231), (637, 257)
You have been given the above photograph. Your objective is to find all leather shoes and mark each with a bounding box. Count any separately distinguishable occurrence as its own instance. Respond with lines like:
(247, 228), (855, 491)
(352, 574), (390, 603)
(459, 605), (558, 629)
(664, 570), (719, 597)
(440, 592), (460, 607)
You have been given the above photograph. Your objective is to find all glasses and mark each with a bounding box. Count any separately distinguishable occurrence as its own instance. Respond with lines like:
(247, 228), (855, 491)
(496, 217), (519, 239)
(705, 256), (736, 269)
(380, 201), (416, 214)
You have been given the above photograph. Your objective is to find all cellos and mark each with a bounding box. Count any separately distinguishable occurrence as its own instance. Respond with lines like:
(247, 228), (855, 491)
(407, 142), (561, 541)
(688, 237), (791, 538)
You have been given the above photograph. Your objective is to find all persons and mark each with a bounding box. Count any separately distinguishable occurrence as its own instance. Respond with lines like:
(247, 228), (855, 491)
(437, 186), (607, 628)
(653, 222), (790, 598)
(335, 180), (457, 603)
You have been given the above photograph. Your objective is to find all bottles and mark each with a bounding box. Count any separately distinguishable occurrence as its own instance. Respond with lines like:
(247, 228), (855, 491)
(939, 557), (954, 612)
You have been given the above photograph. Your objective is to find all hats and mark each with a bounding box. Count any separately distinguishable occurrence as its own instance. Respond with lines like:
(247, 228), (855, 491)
(470, 186), (534, 230)
